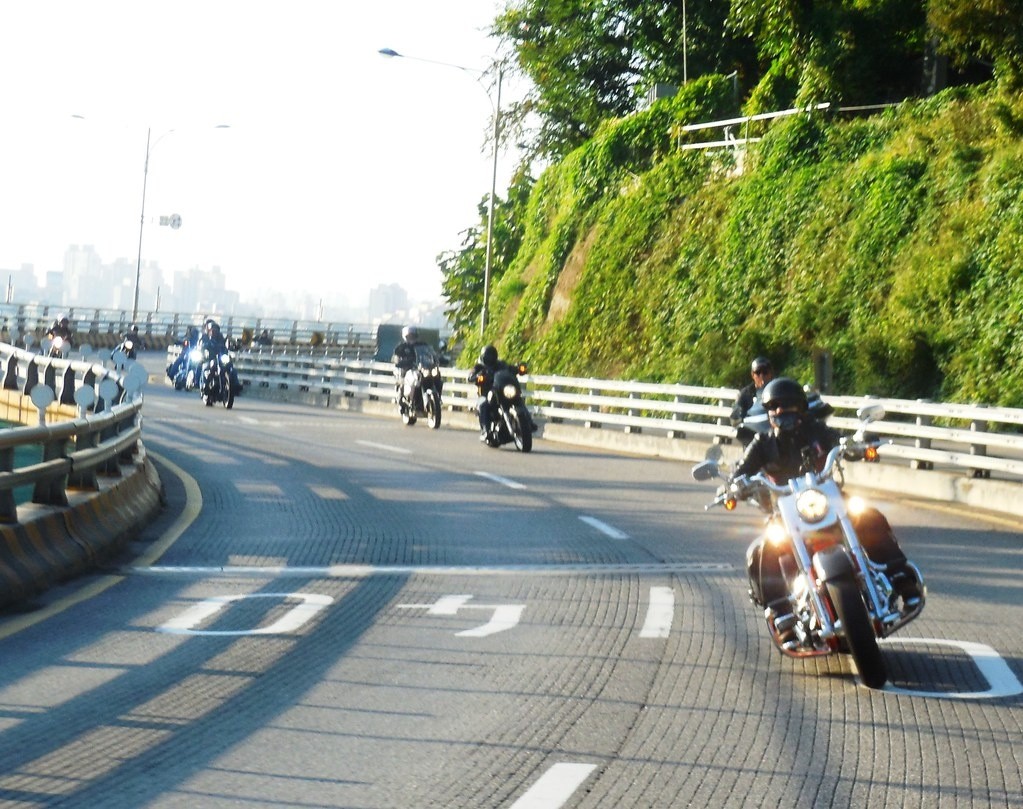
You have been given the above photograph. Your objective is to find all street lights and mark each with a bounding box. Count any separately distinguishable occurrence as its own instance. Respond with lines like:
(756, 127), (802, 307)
(70, 113), (231, 320)
(378, 46), (505, 339)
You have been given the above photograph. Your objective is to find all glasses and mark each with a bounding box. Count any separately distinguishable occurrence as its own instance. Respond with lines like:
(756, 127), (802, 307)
(764, 400), (795, 410)
(754, 368), (770, 375)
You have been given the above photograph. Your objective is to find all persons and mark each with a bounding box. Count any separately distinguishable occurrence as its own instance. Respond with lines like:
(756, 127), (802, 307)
(110, 325), (139, 370)
(45, 318), (72, 357)
(468, 344), (538, 444)
(715, 378), (925, 607)
(729, 356), (829, 442)
(169, 320), (243, 395)
(392, 326), (444, 414)
(258, 329), (270, 345)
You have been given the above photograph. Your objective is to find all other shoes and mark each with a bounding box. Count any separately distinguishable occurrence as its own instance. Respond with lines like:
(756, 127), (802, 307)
(777, 628), (799, 650)
(890, 569), (921, 604)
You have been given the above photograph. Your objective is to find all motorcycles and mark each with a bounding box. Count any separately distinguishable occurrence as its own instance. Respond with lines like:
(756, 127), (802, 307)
(112, 336), (147, 371)
(474, 362), (539, 453)
(714, 383), (837, 514)
(165, 335), (244, 410)
(690, 403), (928, 689)
(394, 340), (448, 430)
(44, 329), (75, 360)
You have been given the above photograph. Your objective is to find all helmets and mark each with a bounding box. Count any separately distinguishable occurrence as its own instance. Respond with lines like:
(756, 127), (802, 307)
(401, 325), (419, 342)
(131, 324), (140, 333)
(751, 357), (771, 372)
(480, 345), (498, 366)
(204, 322), (220, 335)
(761, 378), (809, 413)
